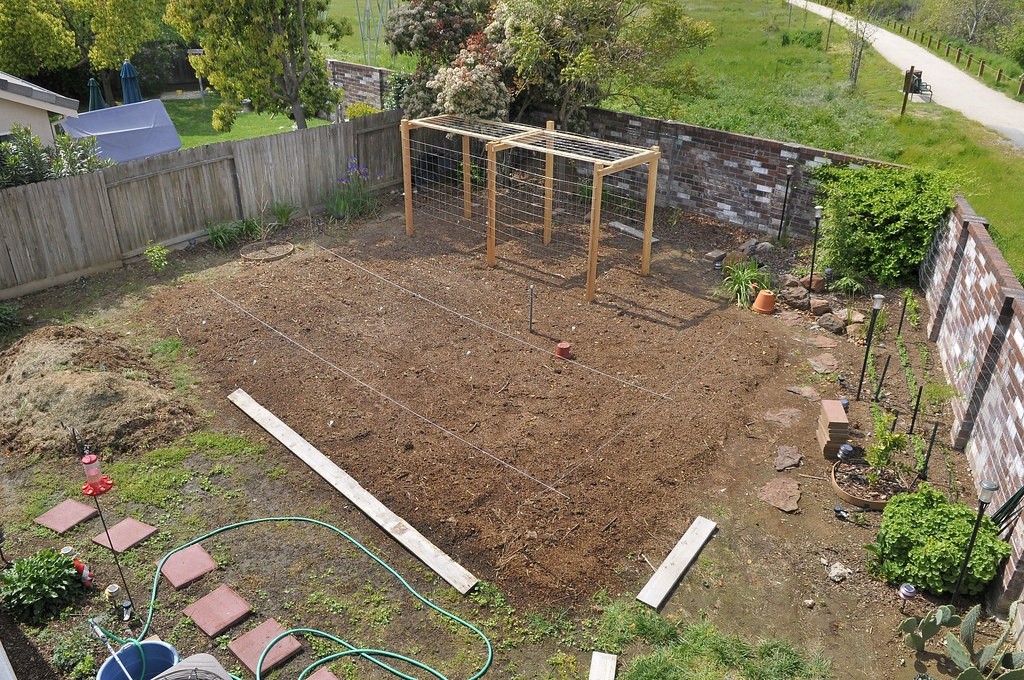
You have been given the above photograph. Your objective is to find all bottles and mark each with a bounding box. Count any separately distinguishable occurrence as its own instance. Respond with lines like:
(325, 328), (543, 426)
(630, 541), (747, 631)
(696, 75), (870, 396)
(82, 455), (103, 486)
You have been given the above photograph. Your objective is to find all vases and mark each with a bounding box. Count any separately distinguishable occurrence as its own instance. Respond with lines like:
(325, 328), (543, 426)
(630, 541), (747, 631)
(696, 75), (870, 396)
(751, 290), (775, 315)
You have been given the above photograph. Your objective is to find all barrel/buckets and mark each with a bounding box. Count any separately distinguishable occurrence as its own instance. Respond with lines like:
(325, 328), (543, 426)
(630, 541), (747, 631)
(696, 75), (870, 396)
(96, 641), (178, 680)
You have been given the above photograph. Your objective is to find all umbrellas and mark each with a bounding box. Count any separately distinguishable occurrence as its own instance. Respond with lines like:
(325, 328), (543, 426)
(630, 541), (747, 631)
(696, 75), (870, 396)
(120, 58), (143, 104)
(87, 78), (106, 112)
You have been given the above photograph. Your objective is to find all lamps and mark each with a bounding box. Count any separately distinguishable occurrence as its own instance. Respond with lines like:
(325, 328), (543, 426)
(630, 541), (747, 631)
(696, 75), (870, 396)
(872, 294), (884, 315)
(81, 454), (113, 496)
(814, 205), (824, 223)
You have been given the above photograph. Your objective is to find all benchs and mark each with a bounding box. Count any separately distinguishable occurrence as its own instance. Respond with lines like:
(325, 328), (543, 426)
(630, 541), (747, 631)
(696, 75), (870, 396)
(910, 73), (932, 103)
